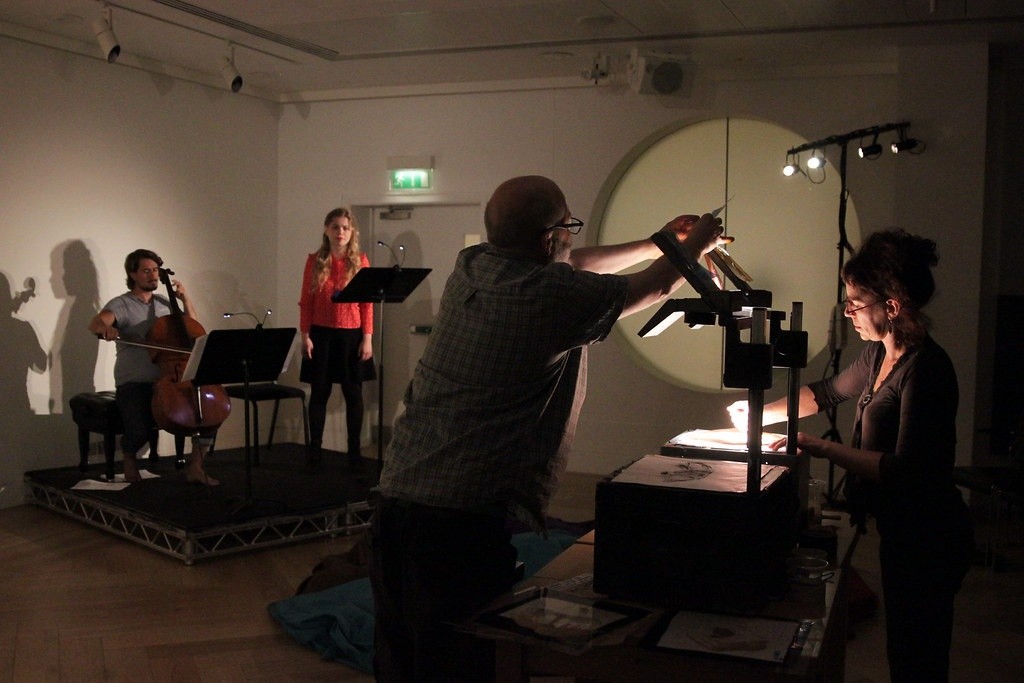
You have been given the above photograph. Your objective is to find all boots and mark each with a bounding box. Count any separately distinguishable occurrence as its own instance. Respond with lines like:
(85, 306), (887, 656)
(306, 444), (322, 472)
(346, 439), (362, 472)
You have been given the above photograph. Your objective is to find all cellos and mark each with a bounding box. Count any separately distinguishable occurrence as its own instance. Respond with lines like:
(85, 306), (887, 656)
(144, 266), (232, 490)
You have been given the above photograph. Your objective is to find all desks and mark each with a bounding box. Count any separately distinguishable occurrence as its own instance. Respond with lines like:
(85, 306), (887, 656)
(459, 502), (858, 683)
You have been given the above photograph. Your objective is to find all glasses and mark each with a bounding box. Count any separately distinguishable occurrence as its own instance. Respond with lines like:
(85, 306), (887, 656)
(840, 297), (904, 315)
(535, 216), (584, 241)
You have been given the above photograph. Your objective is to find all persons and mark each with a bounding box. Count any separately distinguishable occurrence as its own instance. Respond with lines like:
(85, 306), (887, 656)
(370, 176), (735, 683)
(87, 249), (220, 487)
(300, 208), (376, 473)
(727, 228), (958, 683)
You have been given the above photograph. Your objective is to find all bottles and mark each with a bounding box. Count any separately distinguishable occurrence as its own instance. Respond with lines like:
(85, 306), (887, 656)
(807, 478), (825, 530)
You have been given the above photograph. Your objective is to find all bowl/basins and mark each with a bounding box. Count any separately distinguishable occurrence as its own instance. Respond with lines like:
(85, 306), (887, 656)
(794, 548), (827, 561)
(793, 558), (829, 576)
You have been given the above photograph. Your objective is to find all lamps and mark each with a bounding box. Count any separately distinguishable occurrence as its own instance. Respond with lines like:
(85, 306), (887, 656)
(783, 163), (801, 177)
(890, 128), (920, 154)
(806, 156), (826, 170)
(92, 7), (121, 64)
(859, 133), (884, 159)
(219, 46), (243, 93)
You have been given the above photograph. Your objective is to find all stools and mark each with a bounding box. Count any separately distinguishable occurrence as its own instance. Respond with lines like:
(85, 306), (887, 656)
(232, 385), (313, 470)
(69, 393), (160, 481)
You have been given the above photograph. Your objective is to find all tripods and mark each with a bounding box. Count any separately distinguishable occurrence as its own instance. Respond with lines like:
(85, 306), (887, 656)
(192, 327), (296, 525)
(333, 268), (432, 494)
